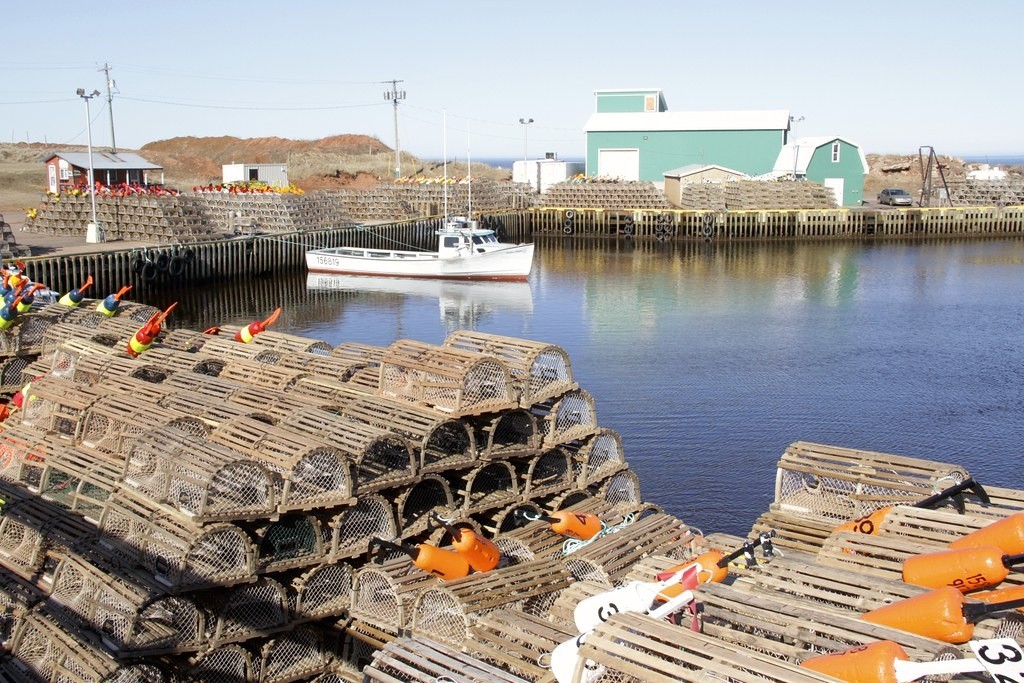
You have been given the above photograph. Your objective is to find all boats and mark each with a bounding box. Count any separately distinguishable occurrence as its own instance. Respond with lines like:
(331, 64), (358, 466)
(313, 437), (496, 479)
(305, 271), (534, 337)
(306, 110), (534, 279)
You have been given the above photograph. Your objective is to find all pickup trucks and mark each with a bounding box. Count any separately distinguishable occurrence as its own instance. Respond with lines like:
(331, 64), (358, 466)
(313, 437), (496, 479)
(877, 188), (913, 206)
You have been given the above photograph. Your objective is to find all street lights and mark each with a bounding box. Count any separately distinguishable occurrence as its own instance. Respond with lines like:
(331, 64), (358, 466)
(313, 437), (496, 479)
(77, 88), (101, 225)
(519, 118), (534, 183)
